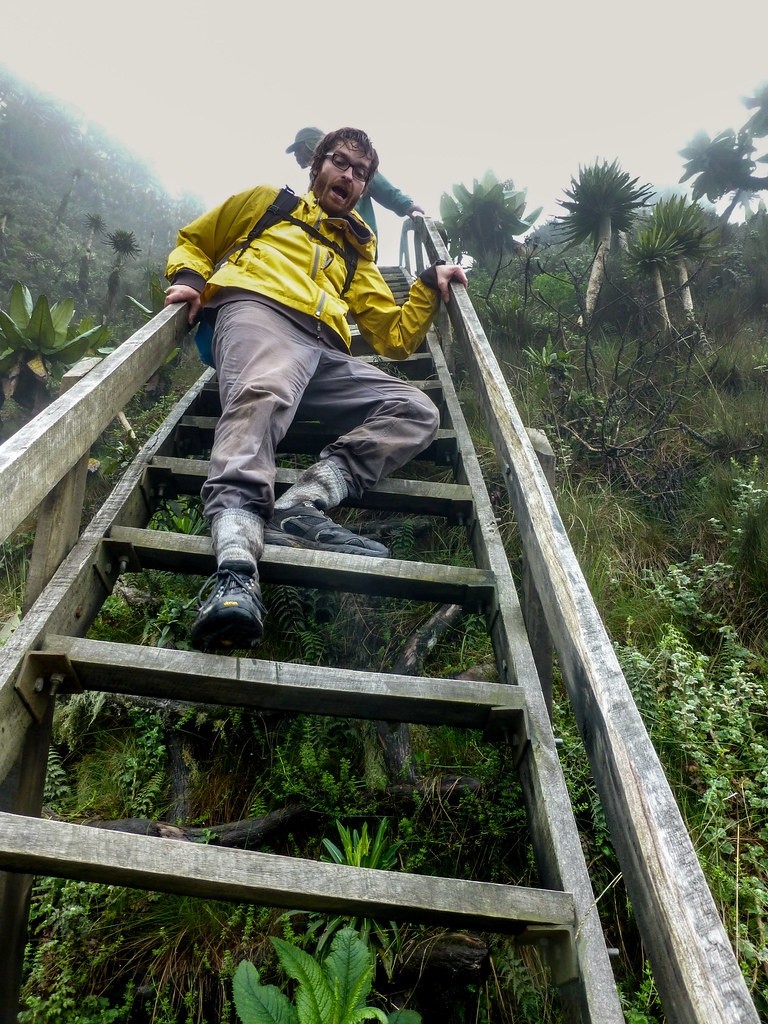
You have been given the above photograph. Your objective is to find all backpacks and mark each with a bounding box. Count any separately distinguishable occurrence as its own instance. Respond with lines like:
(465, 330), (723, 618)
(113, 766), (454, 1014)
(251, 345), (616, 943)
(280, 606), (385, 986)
(194, 309), (218, 367)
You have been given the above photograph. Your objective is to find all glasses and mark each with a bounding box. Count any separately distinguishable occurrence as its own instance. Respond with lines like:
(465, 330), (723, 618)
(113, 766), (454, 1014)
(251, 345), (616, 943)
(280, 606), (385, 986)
(323, 151), (371, 182)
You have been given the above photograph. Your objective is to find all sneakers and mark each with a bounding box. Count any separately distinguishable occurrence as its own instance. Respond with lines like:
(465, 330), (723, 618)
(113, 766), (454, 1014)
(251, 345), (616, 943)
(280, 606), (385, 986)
(264, 500), (391, 558)
(189, 558), (265, 650)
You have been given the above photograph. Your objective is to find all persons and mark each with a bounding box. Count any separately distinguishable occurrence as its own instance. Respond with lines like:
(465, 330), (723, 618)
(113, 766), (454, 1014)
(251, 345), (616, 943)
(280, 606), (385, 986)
(277, 123), (429, 270)
(152, 123), (471, 654)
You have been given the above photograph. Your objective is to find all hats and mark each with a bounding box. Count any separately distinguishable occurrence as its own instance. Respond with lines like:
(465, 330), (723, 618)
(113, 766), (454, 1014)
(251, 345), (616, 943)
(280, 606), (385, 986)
(286, 128), (326, 153)
